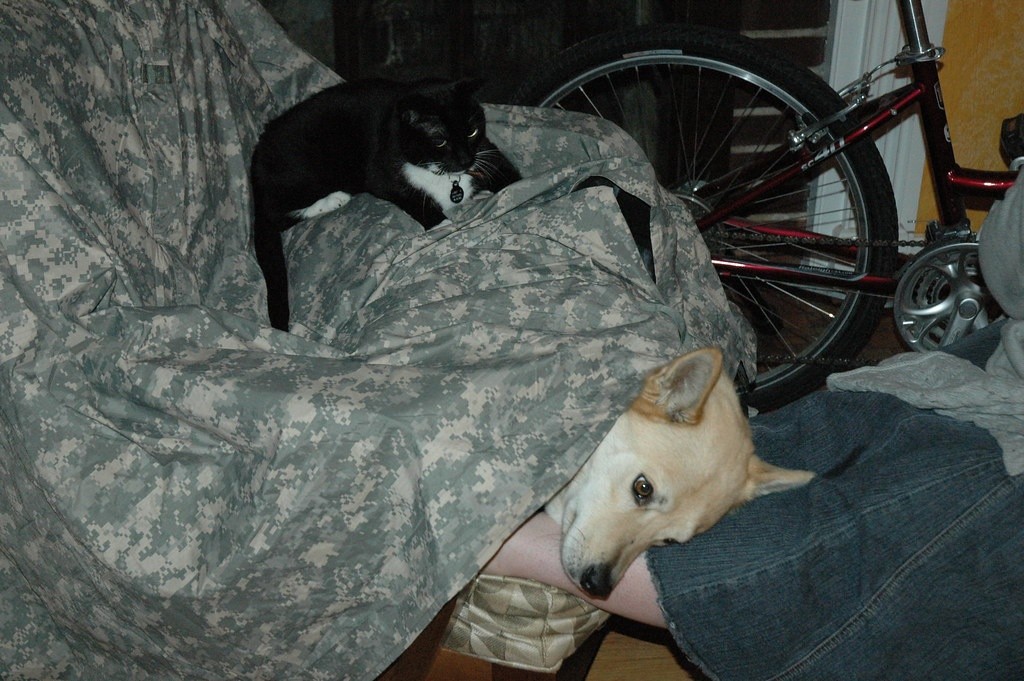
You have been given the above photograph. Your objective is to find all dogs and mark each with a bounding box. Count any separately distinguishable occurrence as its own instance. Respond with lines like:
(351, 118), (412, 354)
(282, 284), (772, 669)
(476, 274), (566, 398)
(545, 345), (817, 602)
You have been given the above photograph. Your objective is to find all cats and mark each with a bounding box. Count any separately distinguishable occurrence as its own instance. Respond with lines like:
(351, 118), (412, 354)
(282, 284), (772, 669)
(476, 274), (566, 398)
(247, 70), (528, 334)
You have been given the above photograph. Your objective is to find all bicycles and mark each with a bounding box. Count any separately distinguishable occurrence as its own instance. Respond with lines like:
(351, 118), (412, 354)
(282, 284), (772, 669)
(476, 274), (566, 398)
(506, 0), (1024, 407)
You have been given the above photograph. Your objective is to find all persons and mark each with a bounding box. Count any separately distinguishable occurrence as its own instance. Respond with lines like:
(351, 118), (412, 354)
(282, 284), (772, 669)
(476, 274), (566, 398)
(475, 163), (1024, 681)
